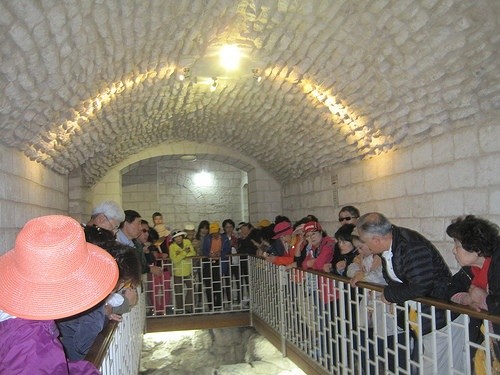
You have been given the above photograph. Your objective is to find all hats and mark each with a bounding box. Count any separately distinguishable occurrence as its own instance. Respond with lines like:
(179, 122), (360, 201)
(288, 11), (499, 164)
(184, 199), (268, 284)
(271, 221), (293, 239)
(209, 222), (220, 234)
(303, 221), (322, 236)
(0, 215), (119, 320)
(169, 229), (187, 241)
(254, 220), (271, 227)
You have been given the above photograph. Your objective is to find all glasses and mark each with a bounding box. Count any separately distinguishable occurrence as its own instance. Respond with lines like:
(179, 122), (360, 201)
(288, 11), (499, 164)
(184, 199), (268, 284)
(141, 228), (150, 233)
(104, 215), (119, 234)
(339, 216), (357, 222)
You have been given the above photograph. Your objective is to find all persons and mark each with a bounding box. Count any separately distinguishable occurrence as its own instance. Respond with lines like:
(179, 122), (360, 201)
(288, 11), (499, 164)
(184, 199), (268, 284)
(442, 216), (500, 375)
(0, 216), (119, 375)
(353, 211), (469, 375)
(52, 241), (140, 361)
(78, 201), (405, 373)
